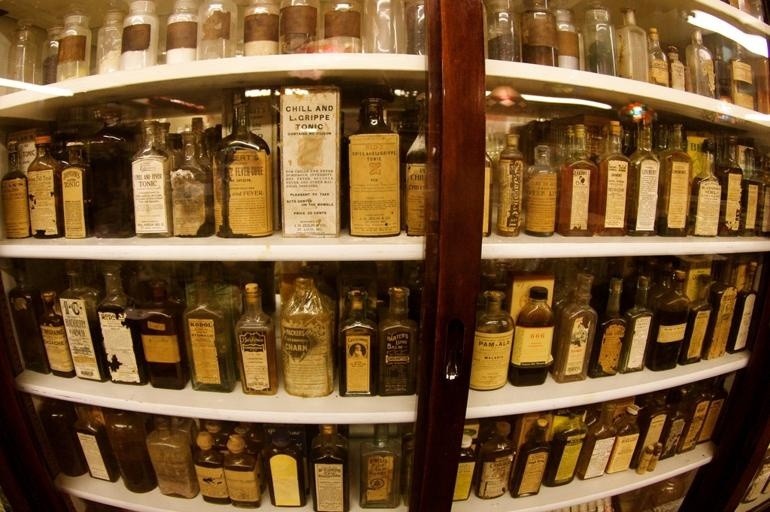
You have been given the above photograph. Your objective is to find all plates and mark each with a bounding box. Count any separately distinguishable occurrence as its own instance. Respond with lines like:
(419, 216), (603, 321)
(1, 0), (770, 512)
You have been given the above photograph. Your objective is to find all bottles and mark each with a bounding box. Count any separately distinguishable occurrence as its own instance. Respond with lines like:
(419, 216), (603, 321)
(15, 256), (760, 393)
(37, 373), (729, 512)
(3, 104), (770, 238)
(7, 1), (770, 116)
